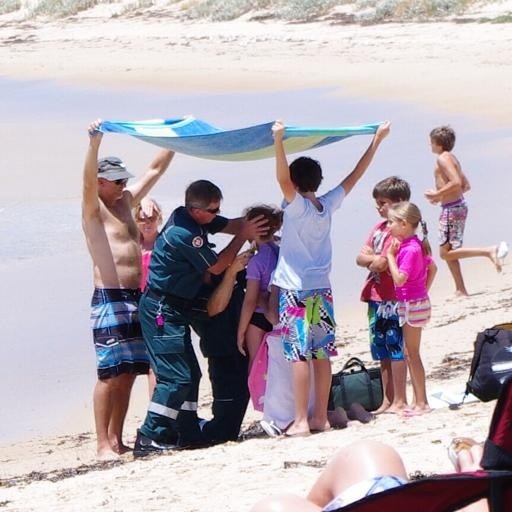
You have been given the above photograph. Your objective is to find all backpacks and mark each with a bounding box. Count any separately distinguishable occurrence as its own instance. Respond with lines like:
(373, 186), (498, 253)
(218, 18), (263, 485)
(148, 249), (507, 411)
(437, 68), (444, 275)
(465, 328), (512, 401)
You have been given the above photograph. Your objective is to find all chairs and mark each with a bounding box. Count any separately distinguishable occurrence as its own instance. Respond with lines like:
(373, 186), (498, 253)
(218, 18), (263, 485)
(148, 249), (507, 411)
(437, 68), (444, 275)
(325, 374), (512, 511)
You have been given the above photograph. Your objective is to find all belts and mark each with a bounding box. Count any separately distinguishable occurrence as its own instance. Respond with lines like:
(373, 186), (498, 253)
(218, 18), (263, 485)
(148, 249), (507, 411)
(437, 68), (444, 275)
(144, 289), (192, 311)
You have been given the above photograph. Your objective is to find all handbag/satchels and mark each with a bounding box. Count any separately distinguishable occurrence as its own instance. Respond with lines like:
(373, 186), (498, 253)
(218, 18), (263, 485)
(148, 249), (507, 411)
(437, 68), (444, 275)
(327, 357), (383, 412)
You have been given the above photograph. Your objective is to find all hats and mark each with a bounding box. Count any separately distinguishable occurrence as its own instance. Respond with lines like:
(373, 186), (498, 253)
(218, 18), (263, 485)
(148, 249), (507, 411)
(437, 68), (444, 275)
(96, 156), (135, 179)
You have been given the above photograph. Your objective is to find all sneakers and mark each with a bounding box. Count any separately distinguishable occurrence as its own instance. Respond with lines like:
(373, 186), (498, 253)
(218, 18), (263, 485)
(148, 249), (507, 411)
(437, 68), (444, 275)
(134, 429), (191, 458)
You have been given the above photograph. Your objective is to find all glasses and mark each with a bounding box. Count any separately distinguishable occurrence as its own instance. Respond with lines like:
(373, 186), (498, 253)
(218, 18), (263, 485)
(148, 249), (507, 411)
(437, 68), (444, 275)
(137, 216), (157, 223)
(113, 180), (129, 184)
(205, 207), (220, 214)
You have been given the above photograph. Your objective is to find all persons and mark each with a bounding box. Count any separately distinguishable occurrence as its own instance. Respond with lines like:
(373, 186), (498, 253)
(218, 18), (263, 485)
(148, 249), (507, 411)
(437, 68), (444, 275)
(421, 123), (505, 303)
(84, 116), (392, 462)
(354, 175), (411, 415)
(382, 200), (438, 415)
(305, 436), (489, 512)
